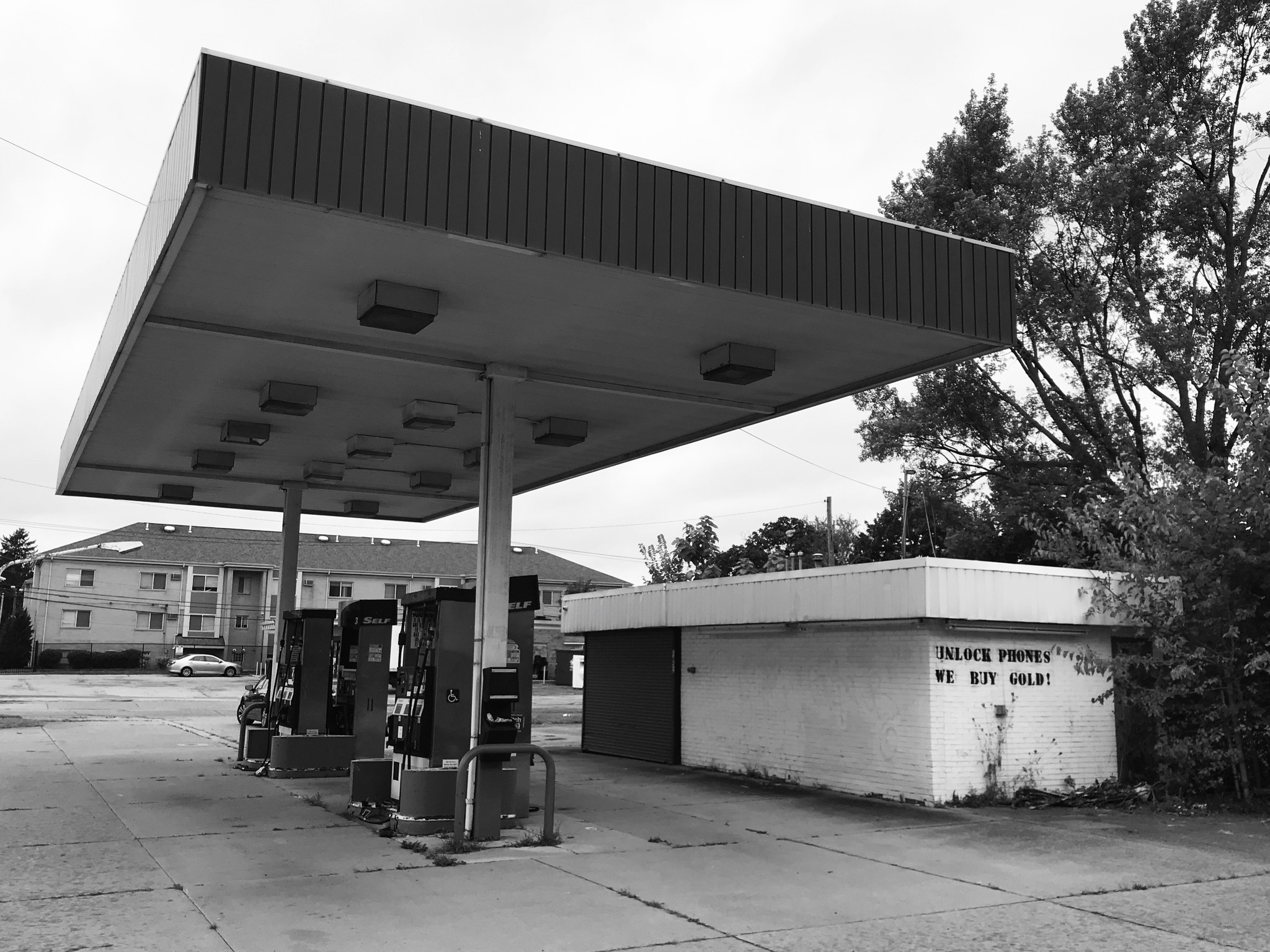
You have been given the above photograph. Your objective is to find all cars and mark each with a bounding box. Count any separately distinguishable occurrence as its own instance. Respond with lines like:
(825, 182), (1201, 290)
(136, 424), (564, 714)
(166, 653), (244, 678)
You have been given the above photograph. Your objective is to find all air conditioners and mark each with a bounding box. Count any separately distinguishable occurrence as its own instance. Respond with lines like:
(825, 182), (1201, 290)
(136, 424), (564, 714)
(170, 573), (181, 580)
(423, 585), (432, 590)
(167, 613), (177, 620)
(304, 580), (314, 586)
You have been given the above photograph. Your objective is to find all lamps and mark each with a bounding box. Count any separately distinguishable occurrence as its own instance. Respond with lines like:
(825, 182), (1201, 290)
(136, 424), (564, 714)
(158, 484), (194, 504)
(409, 470), (452, 493)
(191, 450), (235, 475)
(533, 417), (588, 447)
(700, 342), (777, 385)
(357, 281), (439, 335)
(344, 500), (379, 518)
(347, 435), (394, 462)
(463, 448), (481, 469)
(303, 460), (345, 484)
(259, 381), (318, 417)
(697, 614), (1087, 636)
(220, 420), (271, 446)
(402, 400), (458, 432)
